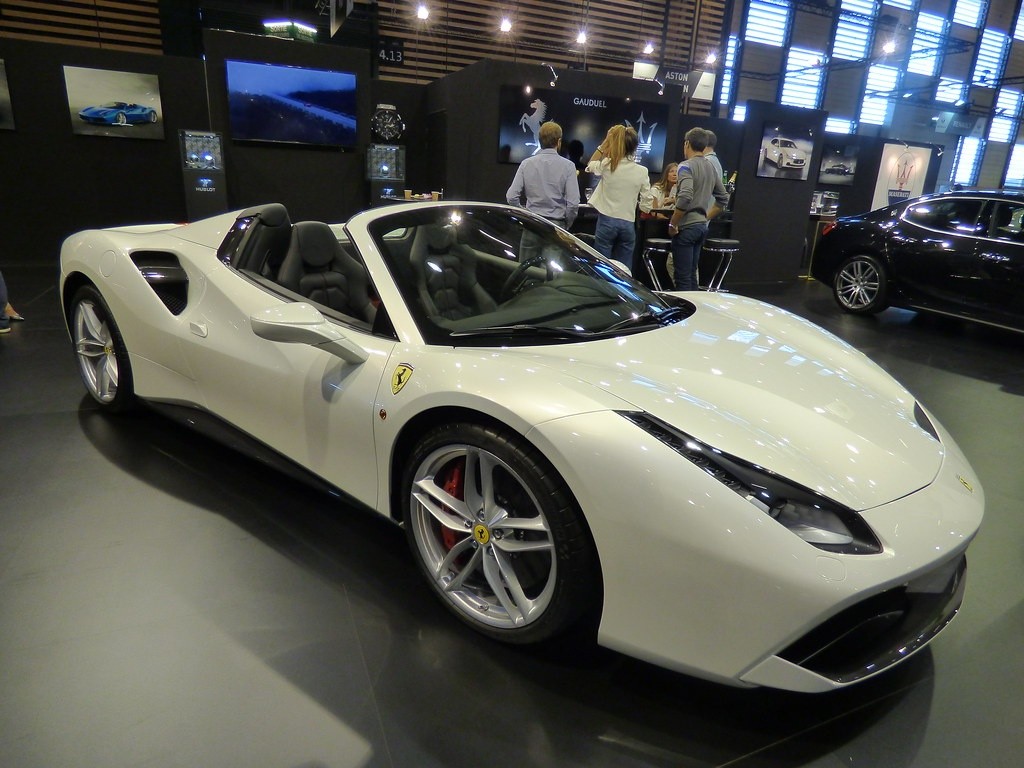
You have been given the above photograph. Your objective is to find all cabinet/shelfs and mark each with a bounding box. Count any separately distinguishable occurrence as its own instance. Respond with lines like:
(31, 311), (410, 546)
(367, 145), (407, 211)
(178, 128), (227, 222)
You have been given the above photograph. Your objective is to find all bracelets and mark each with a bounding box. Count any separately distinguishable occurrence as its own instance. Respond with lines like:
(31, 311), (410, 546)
(668, 223), (677, 229)
(596, 145), (604, 153)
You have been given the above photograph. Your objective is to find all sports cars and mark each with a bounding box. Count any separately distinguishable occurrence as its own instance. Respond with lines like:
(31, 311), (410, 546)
(78, 100), (157, 125)
(59, 200), (986, 695)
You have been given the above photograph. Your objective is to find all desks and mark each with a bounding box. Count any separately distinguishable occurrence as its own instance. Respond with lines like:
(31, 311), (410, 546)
(386, 193), (443, 203)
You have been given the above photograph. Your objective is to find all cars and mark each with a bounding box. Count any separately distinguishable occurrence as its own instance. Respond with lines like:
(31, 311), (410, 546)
(763, 137), (807, 170)
(809, 185), (1024, 337)
(825, 163), (852, 176)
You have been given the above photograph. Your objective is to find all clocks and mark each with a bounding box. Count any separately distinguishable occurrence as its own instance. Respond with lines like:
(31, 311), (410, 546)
(370, 109), (405, 144)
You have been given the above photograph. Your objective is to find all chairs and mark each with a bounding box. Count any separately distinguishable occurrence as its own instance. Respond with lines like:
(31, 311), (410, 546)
(276, 220), (377, 326)
(408, 222), (498, 331)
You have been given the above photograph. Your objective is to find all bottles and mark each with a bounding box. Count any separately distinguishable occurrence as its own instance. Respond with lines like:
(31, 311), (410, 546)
(722, 170), (737, 193)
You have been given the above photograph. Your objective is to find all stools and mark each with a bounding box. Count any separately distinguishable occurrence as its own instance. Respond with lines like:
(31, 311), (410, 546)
(641, 238), (673, 292)
(698, 238), (741, 295)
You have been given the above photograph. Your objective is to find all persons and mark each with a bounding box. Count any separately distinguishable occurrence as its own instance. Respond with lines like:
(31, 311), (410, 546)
(669, 126), (730, 290)
(504, 119), (581, 266)
(650, 163), (679, 208)
(585, 124), (654, 273)
(666, 130), (723, 290)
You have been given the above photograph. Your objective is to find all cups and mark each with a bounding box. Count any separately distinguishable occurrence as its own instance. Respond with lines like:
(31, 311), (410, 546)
(432, 191), (439, 202)
(404, 190), (412, 200)
